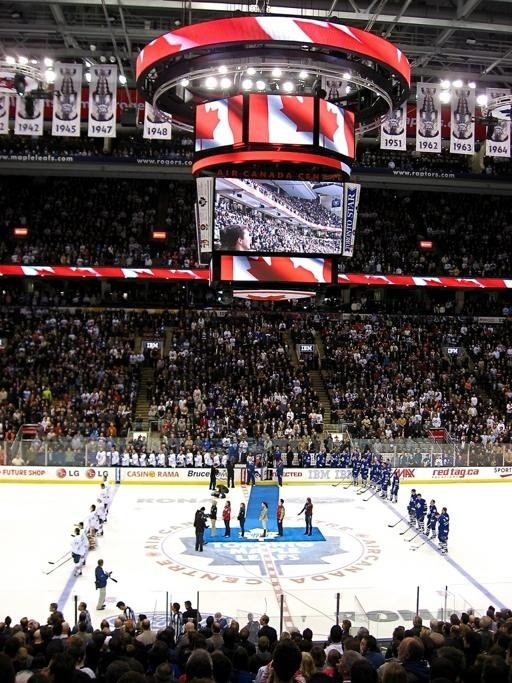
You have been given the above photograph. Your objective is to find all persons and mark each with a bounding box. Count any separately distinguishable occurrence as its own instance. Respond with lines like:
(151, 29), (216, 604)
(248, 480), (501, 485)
(194, 511), (209, 552)
(275, 498), (286, 538)
(1, 598), (512, 682)
(346, 453), (450, 555)
(93, 558), (112, 610)
(69, 475), (112, 577)
(297, 496), (314, 536)
(221, 500), (231, 537)
(258, 501), (270, 537)
(208, 499), (218, 537)
(1, 170), (512, 496)
(195, 506), (208, 545)
(237, 502), (246, 538)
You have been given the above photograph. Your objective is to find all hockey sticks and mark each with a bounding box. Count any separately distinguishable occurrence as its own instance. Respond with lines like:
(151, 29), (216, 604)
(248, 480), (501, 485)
(332, 472), (381, 501)
(388, 514), (435, 550)
(42, 557), (71, 575)
(48, 551), (72, 564)
